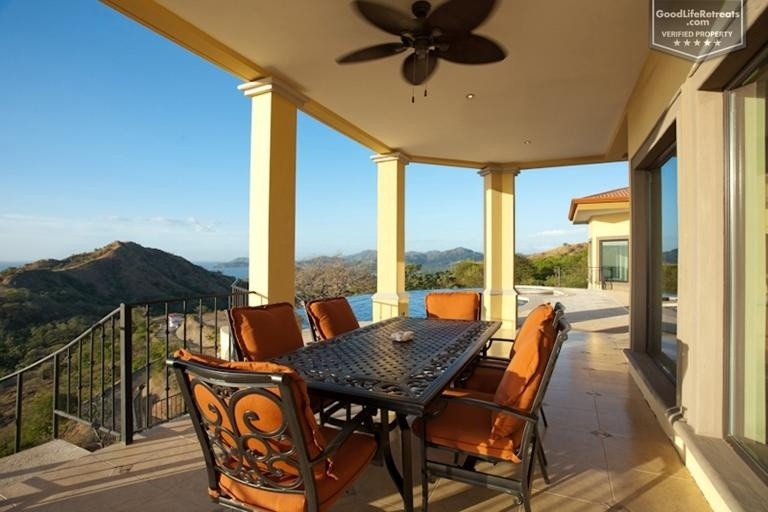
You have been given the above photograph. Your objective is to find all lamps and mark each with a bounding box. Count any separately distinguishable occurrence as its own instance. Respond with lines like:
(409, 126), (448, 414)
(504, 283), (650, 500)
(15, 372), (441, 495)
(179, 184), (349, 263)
(396, 26), (450, 56)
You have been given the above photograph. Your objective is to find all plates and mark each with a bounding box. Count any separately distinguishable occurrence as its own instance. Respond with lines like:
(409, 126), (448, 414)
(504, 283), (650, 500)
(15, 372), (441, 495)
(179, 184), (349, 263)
(390, 332), (414, 341)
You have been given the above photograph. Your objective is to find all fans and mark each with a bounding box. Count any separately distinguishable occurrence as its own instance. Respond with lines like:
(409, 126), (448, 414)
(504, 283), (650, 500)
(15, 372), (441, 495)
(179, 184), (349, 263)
(332, 1), (510, 84)
